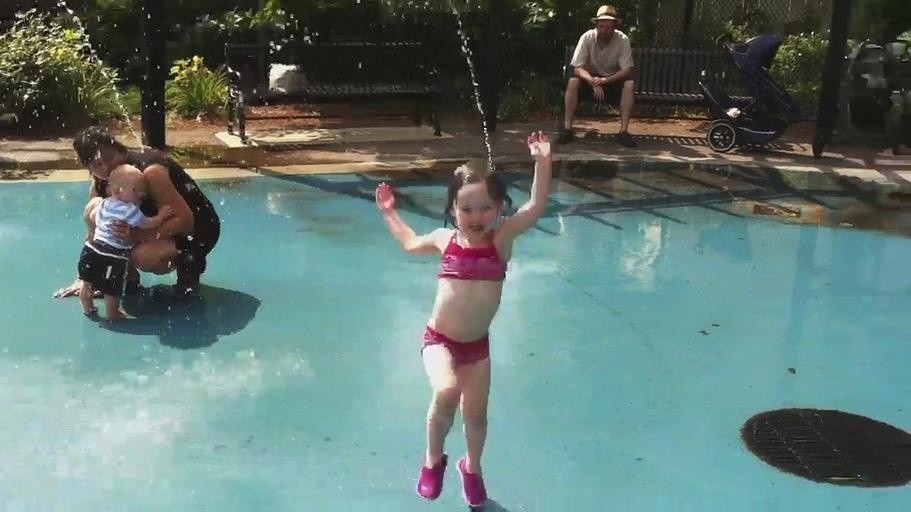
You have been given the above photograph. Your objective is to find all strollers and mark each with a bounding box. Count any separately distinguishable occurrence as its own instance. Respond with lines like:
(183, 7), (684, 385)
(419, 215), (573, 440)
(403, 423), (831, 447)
(696, 34), (825, 160)
(809, 37), (911, 158)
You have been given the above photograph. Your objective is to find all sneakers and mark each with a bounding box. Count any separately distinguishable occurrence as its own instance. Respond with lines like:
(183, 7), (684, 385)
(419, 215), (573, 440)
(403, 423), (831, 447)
(558, 129), (573, 143)
(416, 453), (448, 500)
(456, 456), (487, 507)
(617, 132), (634, 147)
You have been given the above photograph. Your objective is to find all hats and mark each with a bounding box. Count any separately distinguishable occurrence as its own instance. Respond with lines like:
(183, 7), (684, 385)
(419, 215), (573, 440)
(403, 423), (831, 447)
(590, 5), (623, 25)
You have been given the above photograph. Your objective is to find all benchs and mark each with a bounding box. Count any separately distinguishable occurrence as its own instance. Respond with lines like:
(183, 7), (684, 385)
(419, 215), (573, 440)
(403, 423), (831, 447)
(558, 44), (736, 129)
(222, 23), (448, 144)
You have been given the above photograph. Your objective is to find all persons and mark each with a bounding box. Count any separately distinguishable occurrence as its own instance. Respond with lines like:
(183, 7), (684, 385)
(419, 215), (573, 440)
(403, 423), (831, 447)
(557, 5), (636, 147)
(374, 130), (552, 507)
(54, 126), (220, 298)
(78, 163), (174, 321)
